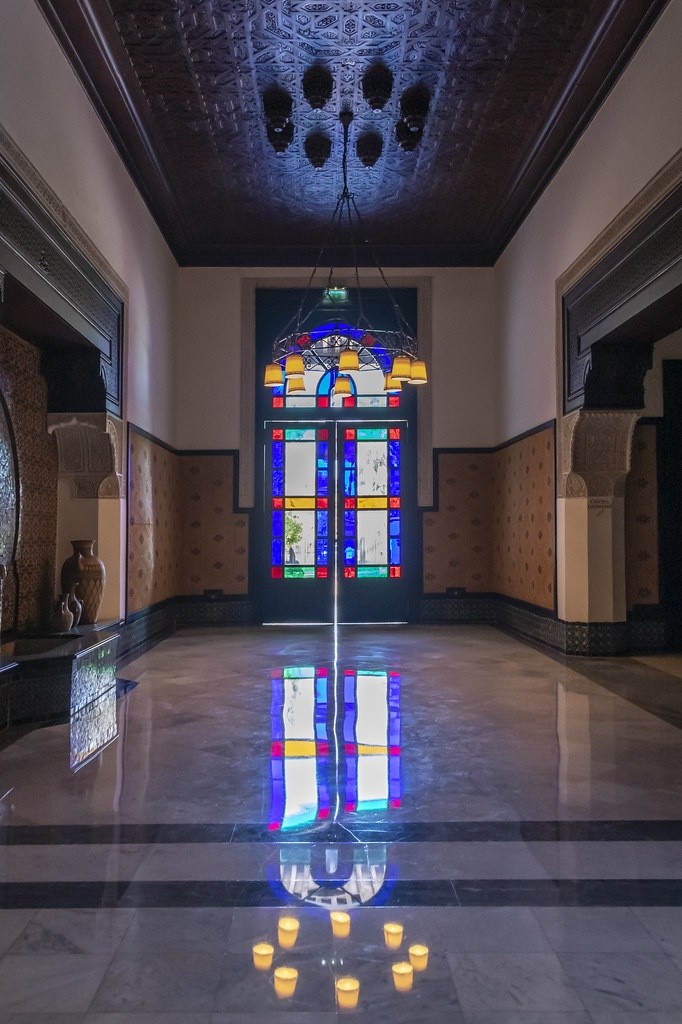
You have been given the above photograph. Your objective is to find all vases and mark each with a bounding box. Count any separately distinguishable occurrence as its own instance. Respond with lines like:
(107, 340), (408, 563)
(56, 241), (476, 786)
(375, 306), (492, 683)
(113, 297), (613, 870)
(61, 540), (105, 625)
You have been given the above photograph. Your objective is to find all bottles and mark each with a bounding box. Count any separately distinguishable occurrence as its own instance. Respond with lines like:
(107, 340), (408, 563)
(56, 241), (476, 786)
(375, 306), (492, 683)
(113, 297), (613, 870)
(61, 540), (106, 625)
(53, 582), (83, 628)
(52, 594), (73, 632)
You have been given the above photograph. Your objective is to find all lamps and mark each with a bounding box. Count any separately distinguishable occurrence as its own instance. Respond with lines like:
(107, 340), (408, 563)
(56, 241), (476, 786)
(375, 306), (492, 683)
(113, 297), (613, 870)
(261, 64), (430, 399)
